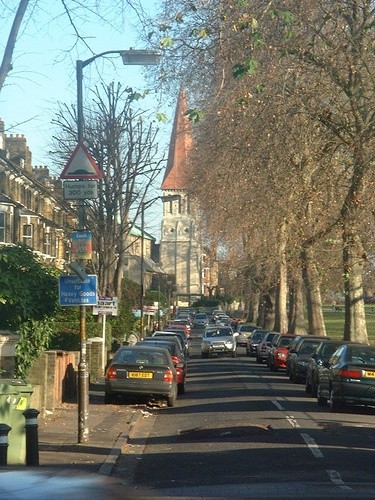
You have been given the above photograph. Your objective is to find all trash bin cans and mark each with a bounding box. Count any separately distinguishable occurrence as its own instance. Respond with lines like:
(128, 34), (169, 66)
(0, 379), (34, 464)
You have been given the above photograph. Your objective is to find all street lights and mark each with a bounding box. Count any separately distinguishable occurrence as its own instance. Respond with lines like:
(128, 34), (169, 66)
(76, 48), (162, 443)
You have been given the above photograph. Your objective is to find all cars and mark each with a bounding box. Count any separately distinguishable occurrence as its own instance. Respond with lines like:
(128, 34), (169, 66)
(257, 331), (281, 363)
(246, 329), (269, 356)
(266, 334), (300, 371)
(305, 339), (365, 397)
(194, 313), (209, 327)
(104, 346), (185, 407)
(315, 344), (375, 412)
(134, 341), (190, 393)
(286, 335), (330, 374)
(206, 308), (260, 347)
(198, 326), (240, 358)
(144, 307), (197, 357)
(289, 338), (331, 383)
